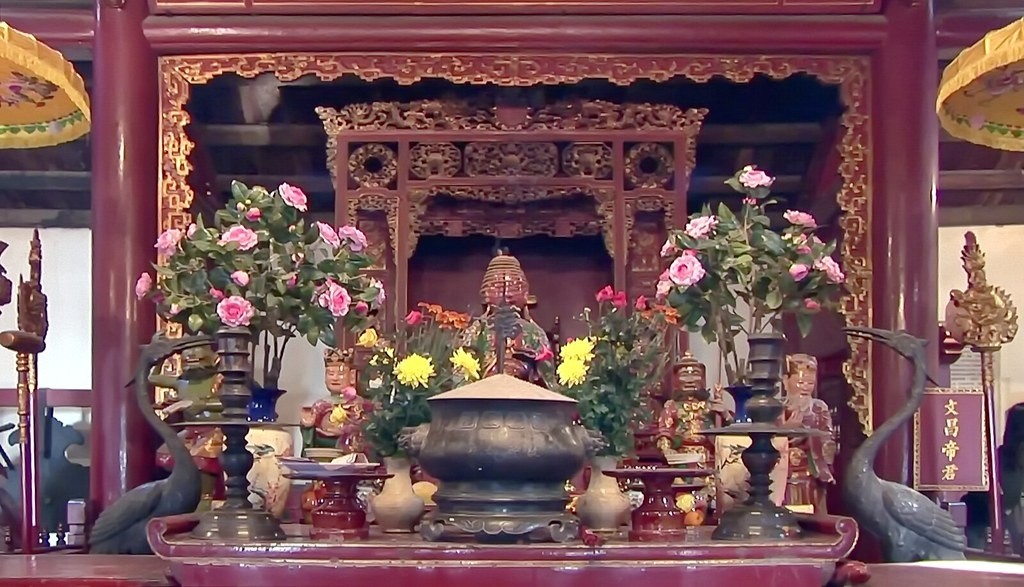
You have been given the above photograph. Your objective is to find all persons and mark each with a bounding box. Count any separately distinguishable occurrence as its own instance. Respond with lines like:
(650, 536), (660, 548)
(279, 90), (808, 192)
(776, 355), (837, 513)
(300, 348), (376, 449)
(155, 322), (222, 473)
(659, 355), (737, 511)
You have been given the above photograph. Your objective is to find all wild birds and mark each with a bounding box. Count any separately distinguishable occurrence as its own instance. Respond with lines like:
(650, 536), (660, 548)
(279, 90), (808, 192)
(88, 337), (218, 555)
(842, 326), (967, 562)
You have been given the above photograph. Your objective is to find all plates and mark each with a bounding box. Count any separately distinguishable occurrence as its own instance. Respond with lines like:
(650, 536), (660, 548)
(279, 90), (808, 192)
(276, 462), (381, 474)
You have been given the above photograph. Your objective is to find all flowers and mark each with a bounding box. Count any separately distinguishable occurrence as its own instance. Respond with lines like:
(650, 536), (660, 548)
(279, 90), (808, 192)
(654, 163), (846, 384)
(136, 181), (388, 390)
(515, 285), (680, 455)
(341, 300), (495, 454)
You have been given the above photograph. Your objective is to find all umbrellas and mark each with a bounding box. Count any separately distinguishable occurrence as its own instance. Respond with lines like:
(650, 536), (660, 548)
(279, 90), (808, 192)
(0, 22), (92, 151)
(935, 17), (1024, 151)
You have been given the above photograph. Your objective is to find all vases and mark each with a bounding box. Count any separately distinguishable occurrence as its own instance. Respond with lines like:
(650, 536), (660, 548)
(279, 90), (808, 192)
(221, 389), (293, 522)
(573, 455), (628, 531)
(368, 455), (424, 532)
(711, 388), (790, 520)
